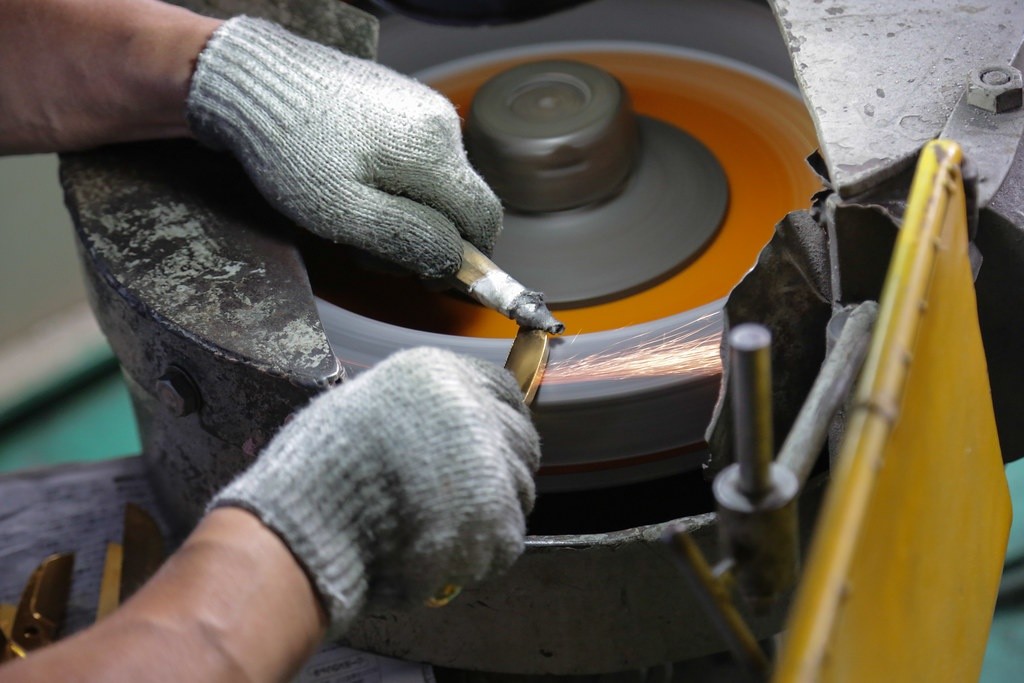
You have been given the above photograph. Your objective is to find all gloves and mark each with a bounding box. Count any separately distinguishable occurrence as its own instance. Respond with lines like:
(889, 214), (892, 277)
(186, 15), (506, 285)
(205, 344), (545, 648)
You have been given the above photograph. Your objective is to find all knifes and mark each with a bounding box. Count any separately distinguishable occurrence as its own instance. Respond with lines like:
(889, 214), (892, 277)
(425, 325), (551, 606)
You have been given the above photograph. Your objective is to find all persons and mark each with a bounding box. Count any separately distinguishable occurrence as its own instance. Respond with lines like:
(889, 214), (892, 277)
(0, 1), (541, 683)
